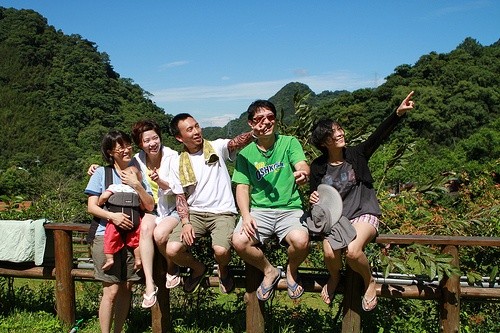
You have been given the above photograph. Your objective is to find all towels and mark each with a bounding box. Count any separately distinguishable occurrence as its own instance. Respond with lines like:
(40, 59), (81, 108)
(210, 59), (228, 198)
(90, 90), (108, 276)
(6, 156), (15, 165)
(178, 139), (220, 190)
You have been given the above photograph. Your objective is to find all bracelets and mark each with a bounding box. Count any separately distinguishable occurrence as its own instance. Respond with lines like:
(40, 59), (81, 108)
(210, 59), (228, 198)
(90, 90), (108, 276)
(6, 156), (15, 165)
(182, 221), (192, 228)
(251, 130), (259, 139)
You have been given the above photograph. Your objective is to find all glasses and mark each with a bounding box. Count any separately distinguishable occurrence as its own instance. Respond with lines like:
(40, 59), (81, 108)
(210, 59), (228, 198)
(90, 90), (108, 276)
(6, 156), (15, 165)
(253, 115), (276, 124)
(112, 143), (132, 153)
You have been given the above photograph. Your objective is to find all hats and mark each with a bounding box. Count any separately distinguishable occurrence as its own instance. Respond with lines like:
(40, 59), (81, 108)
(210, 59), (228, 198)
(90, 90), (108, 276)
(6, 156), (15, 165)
(306, 183), (357, 251)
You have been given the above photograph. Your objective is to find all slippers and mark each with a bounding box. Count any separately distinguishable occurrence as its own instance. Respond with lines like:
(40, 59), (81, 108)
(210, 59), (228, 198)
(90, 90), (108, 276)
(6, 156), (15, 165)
(256, 268), (305, 301)
(182, 263), (236, 295)
(361, 276), (377, 311)
(141, 287), (159, 308)
(321, 273), (340, 305)
(165, 266), (181, 288)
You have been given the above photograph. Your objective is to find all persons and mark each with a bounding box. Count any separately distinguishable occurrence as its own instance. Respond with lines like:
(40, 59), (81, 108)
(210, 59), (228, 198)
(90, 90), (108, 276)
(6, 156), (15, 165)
(84, 130), (155, 333)
(230, 99), (313, 302)
(164, 112), (268, 295)
(97, 166), (147, 271)
(308, 89), (416, 313)
(86, 120), (183, 309)
